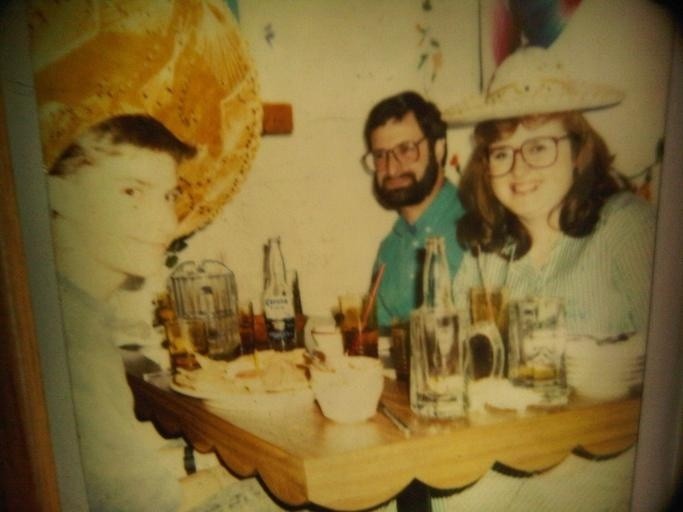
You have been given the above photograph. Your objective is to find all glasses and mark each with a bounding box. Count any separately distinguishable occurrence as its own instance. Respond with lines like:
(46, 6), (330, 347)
(362, 135), (428, 174)
(481, 131), (571, 177)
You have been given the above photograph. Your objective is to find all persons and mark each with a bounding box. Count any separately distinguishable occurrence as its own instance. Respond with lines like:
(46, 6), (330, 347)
(362, 89), (465, 332)
(439, 47), (655, 512)
(27, 0), (265, 511)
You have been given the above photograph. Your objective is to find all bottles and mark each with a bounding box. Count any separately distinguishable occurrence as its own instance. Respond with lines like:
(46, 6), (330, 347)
(260, 237), (299, 352)
(409, 236), (466, 419)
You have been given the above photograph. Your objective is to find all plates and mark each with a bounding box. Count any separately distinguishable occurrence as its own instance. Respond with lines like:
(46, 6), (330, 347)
(168, 367), (306, 400)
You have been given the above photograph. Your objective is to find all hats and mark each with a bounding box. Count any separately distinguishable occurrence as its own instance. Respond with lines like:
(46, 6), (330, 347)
(31, 1), (264, 244)
(442, 44), (625, 124)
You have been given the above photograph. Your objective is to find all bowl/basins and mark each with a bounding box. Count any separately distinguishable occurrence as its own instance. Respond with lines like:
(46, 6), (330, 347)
(312, 356), (387, 423)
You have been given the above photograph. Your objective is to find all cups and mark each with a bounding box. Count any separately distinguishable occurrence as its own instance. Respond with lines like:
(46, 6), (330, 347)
(338, 292), (380, 357)
(509, 296), (571, 407)
(391, 323), (413, 383)
(163, 320), (211, 370)
(471, 286), (514, 378)
